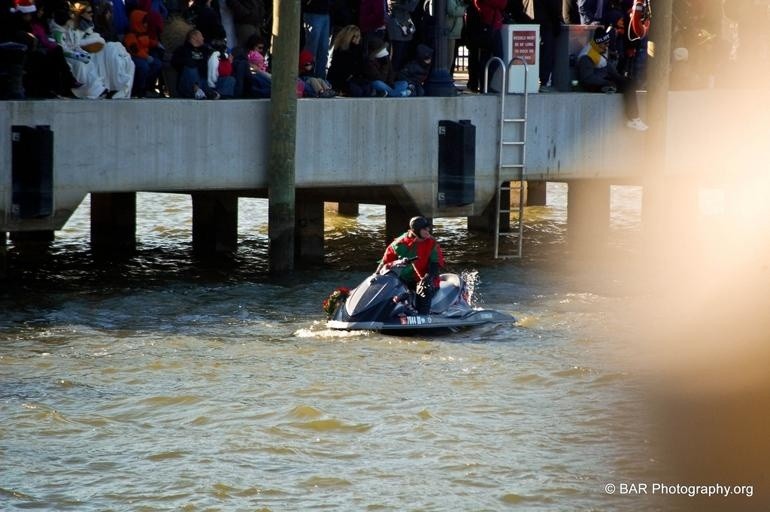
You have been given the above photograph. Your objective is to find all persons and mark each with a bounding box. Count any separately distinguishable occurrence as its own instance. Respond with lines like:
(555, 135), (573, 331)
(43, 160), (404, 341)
(0, 1), (770, 102)
(575, 26), (650, 131)
(382, 215), (445, 315)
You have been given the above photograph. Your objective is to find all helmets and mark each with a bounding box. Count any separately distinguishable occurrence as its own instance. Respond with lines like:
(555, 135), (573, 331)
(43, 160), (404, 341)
(409, 216), (433, 239)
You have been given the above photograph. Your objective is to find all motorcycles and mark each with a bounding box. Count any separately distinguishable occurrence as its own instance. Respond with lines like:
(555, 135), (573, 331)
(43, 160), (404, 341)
(327, 255), (518, 336)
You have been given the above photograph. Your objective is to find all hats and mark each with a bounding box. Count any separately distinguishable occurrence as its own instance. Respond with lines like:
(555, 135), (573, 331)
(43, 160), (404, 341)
(592, 26), (612, 46)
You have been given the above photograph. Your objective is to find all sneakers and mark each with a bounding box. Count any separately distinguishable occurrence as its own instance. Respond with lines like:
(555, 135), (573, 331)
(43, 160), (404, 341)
(317, 87), (338, 98)
(539, 85), (550, 94)
(195, 89), (222, 100)
(371, 87), (411, 98)
(626, 118), (650, 133)
